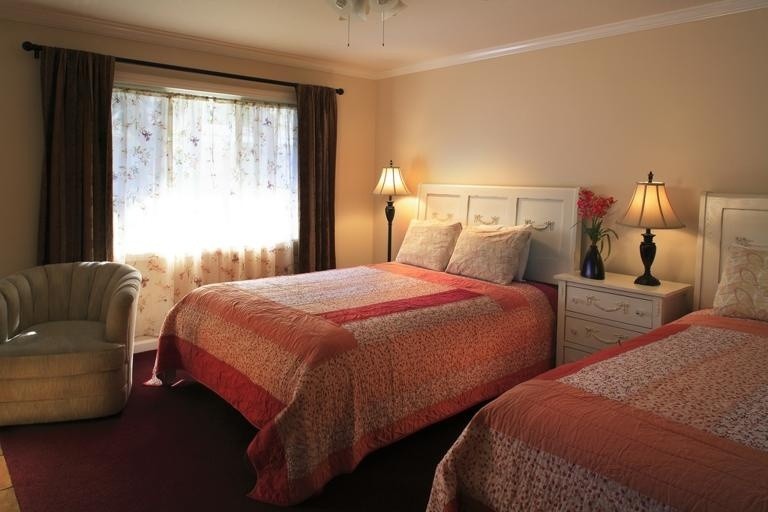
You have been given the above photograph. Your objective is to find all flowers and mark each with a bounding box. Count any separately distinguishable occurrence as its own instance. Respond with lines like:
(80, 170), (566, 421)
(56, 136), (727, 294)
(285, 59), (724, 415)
(576, 187), (619, 258)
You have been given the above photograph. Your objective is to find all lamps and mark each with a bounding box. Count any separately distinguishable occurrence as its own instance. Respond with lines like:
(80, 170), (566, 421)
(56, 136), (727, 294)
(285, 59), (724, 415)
(617, 170), (686, 286)
(372, 159), (412, 262)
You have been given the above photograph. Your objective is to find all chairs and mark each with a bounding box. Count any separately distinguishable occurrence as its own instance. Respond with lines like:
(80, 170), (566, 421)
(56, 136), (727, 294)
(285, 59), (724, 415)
(0, 260), (143, 426)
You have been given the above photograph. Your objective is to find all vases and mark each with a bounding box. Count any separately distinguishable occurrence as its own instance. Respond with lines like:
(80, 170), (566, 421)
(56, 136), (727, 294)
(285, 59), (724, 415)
(580, 243), (606, 279)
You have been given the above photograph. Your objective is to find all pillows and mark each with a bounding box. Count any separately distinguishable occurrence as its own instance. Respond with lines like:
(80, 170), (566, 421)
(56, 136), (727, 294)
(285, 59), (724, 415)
(445, 224), (531, 285)
(480, 224), (532, 282)
(712, 241), (767, 321)
(395, 219), (463, 272)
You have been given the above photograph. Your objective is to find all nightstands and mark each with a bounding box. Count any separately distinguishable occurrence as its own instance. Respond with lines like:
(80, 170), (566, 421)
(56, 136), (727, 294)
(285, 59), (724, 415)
(554, 270), (692, 365)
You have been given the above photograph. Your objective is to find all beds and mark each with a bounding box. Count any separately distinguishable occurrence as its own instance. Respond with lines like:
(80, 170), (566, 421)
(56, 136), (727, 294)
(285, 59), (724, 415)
(425, 191), (767, 511)
(155, 183), (577, 506)
